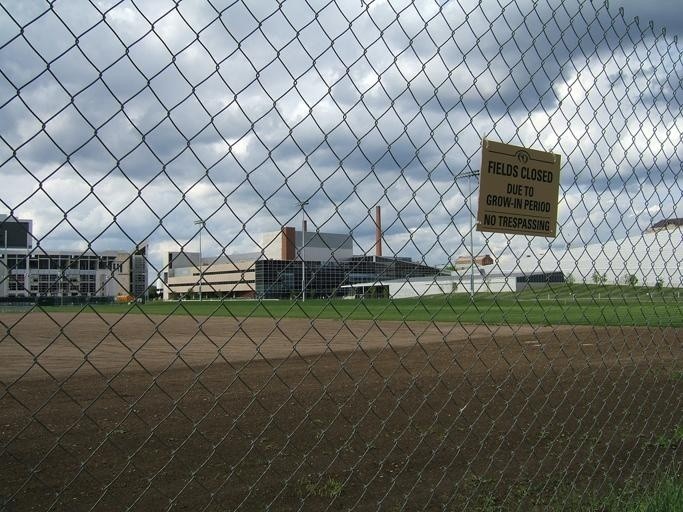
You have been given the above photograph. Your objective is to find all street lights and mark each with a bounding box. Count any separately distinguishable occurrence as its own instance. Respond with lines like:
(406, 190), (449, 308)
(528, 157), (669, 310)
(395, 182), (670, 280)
(293, 199), (311, 301)
(194, 219), (208, 302)
(457, 169), (481, 303)
